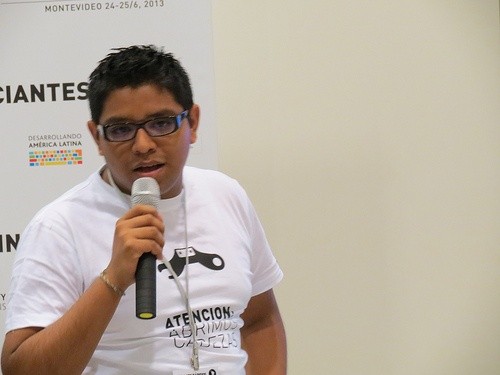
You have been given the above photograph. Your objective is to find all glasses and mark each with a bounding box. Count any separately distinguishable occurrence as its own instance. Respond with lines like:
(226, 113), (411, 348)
(96, 109), (189, 143)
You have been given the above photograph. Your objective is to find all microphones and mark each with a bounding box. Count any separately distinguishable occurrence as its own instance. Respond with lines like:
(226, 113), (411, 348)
(129, 177), (162, 321)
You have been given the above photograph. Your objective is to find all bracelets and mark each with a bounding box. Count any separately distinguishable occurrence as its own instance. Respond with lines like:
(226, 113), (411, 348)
(101, 270), (127, 296)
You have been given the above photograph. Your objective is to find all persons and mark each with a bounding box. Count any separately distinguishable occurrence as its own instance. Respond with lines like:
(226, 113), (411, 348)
(1, 45), (291, 375)
